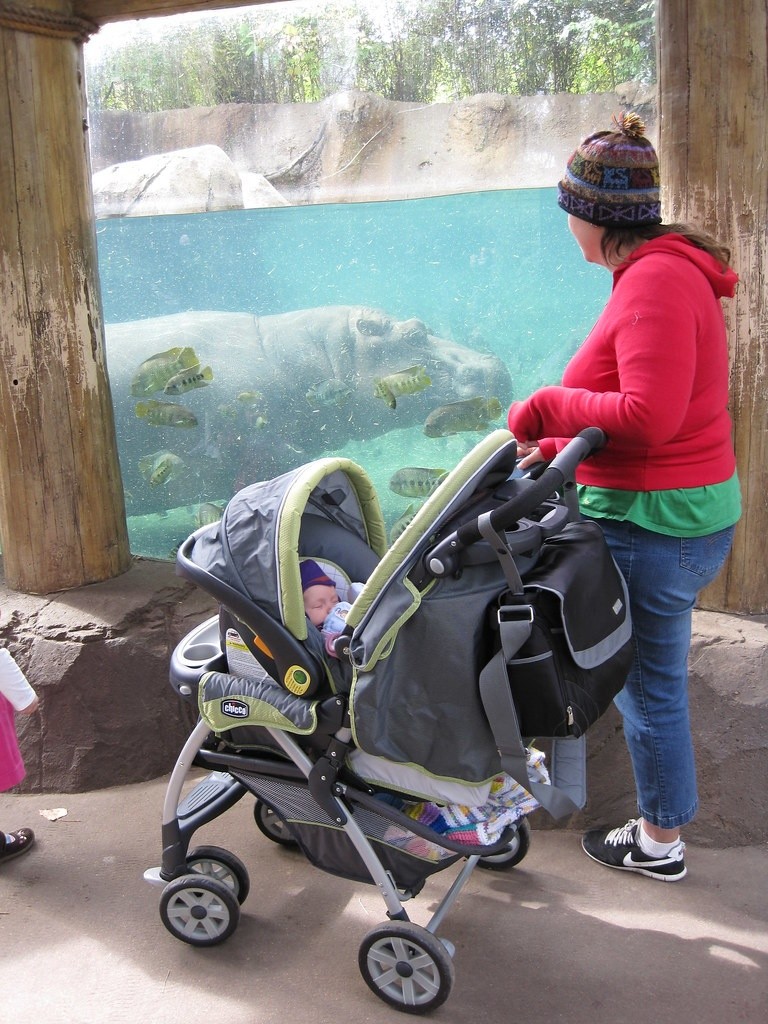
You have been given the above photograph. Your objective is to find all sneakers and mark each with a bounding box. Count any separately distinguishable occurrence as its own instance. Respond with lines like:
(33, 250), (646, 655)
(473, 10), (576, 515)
(582, 815), (687, 881)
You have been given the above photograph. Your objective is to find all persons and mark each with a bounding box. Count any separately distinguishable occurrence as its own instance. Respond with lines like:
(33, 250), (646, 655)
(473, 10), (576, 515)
(300, 558), (353, 638)
(0, 647), (39, 863)
(505, 105), (747, 882)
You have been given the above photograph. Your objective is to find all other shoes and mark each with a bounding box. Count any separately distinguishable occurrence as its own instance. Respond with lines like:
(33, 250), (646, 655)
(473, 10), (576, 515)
(0, 828), (34, 863)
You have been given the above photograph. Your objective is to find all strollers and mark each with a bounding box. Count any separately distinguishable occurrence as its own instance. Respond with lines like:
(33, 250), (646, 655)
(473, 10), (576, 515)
(156, 428), (607, 1017)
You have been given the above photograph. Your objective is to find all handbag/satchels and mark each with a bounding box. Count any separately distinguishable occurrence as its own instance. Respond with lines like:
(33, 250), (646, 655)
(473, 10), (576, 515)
(477, 509), (635, 739)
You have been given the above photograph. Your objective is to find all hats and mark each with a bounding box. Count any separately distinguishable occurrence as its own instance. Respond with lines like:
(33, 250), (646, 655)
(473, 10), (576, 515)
(556, 109), (663, 228)
(300, 559), (336, 594)
